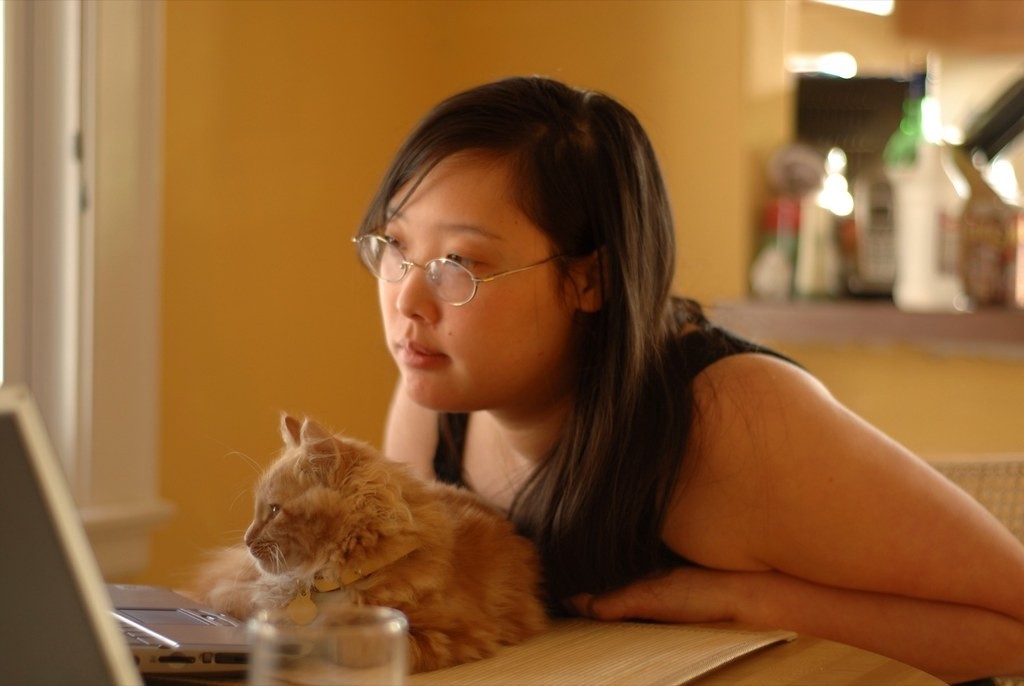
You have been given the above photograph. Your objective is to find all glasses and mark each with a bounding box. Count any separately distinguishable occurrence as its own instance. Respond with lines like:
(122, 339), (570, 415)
(352, 231), (577, 306)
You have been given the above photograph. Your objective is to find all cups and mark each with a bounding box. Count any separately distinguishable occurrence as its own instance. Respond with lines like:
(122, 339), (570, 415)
(246, 606), (406, 686)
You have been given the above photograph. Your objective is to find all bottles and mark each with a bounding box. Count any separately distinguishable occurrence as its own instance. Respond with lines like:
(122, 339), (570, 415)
(889, 106), (967, 312)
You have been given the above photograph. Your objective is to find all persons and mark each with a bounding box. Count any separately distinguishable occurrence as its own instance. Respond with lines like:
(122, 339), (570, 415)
(356, 76), (1024, 686)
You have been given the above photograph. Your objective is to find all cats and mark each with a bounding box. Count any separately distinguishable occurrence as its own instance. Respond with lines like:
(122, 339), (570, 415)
(198, 413), (554, 677)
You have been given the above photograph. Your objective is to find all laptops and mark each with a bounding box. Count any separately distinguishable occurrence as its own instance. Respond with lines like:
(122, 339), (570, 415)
(1, 392), (250, 685)
